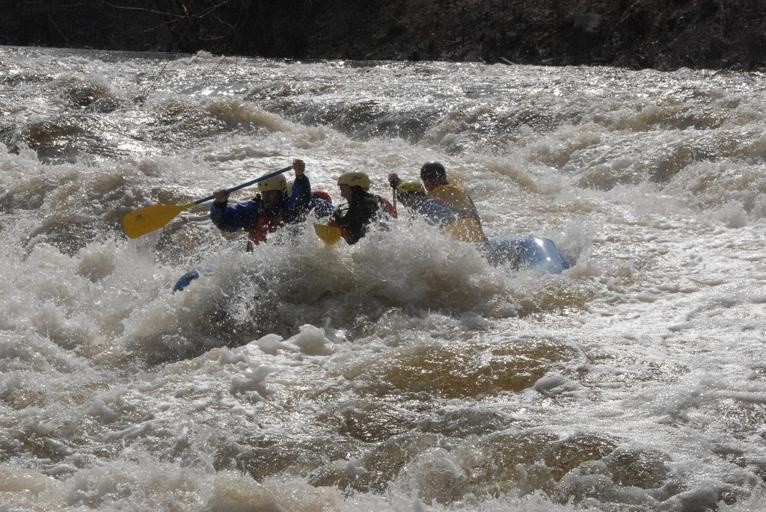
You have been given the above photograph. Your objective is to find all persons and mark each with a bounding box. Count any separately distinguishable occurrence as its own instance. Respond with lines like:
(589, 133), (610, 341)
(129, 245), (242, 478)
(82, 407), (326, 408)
(209, 157), (488, 253)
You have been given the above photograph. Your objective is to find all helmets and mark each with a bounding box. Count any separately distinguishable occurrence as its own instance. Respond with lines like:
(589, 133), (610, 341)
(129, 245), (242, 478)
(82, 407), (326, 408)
(420, 160), (446, 180)
(337, 171), (370, 193)
(396, 181), (426, 202)
(258, 171), (287, 196)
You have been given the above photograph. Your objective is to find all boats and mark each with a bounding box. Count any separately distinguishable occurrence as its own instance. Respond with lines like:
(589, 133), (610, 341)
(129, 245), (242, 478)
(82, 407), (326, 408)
(171, 233), (570, 295)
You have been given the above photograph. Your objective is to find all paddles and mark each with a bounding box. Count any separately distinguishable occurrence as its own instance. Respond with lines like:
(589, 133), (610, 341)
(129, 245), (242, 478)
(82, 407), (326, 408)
(315, 224), (341, 247)
(121, 166), (294, 238)
